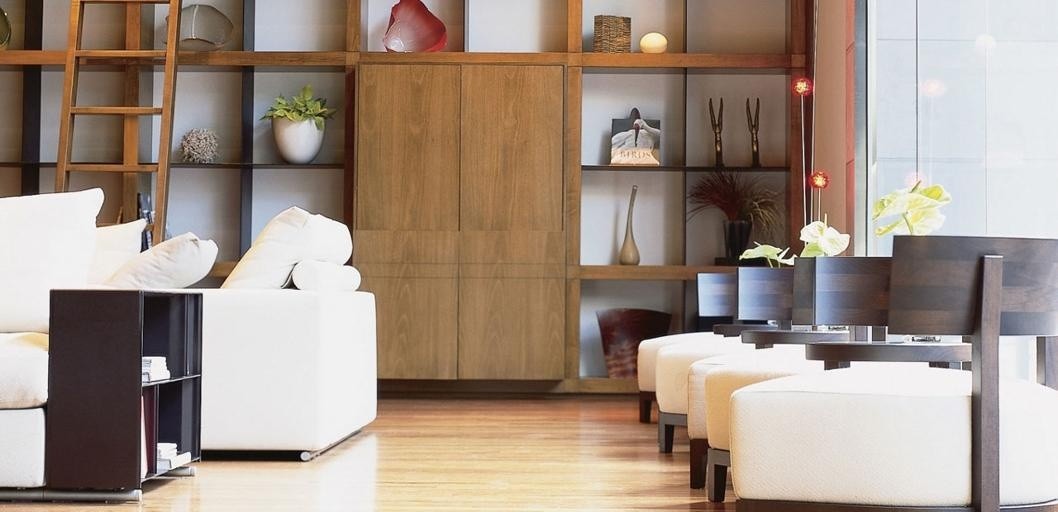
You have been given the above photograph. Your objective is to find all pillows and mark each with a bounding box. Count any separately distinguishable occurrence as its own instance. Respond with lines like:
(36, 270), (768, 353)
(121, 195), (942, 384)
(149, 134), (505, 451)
(97, 218), (148, 290)
(102, 231), (218, 288)
(0, 187), (104, 333)
(292, 260), (362, 291)
(218, 206), (355, 290)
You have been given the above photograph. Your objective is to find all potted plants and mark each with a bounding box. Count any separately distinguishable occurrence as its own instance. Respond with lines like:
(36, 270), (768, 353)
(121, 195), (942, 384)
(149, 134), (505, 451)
(800, 213), (851, 330)
(872, 180), (951, 343)
(739, 240), (798, 327)
(687, 172), (787, 258)
(259, 82), (338, 165)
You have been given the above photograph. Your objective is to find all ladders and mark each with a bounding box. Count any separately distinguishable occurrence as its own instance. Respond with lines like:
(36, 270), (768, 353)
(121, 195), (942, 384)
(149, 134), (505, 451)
(53, 0), (182, 247)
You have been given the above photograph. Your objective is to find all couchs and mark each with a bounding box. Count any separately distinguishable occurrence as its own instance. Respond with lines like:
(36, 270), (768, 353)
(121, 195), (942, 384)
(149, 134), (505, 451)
(0, 187), (219, 487)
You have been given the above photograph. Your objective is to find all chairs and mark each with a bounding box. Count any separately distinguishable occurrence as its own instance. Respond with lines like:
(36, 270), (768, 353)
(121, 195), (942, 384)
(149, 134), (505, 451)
(637, 237), (1058, 511)
(151, 287), (377, 460)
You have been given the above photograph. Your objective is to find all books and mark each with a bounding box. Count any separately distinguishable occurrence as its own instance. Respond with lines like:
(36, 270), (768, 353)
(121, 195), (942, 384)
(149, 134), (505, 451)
(142, 354), (171, 383)
(155, 441), (193, 473)
(136, 190), (154, 252)
(147, 209), (168, 246)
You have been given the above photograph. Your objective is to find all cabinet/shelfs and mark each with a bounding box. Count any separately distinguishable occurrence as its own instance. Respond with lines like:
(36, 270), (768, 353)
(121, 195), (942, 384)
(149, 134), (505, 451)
(566, 67), (806, 393)
(1, 66), (353, 277)
(353, 64), (567, 231)
(353, 231), (566, 393)
(44, 290), (203, 501)
(0, 0), (806, 67)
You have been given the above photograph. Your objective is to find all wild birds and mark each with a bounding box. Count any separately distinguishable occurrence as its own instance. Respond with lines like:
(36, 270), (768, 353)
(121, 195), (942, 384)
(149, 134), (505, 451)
(611, 118), (659, 148)
